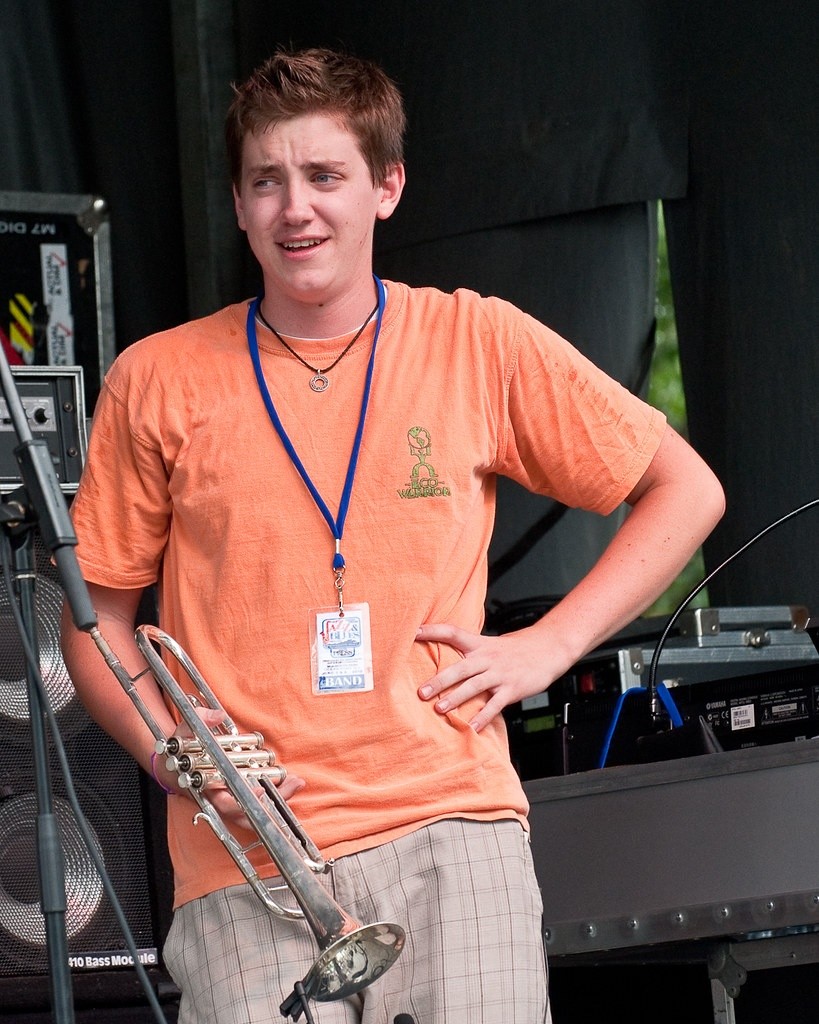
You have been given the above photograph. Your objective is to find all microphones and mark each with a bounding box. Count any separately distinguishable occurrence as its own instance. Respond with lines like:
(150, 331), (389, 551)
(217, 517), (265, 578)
(394, 1013), (415, 1024)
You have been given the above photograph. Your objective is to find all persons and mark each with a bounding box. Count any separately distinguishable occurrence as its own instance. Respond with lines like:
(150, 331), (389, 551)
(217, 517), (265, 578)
(59, 48), (727, 1024)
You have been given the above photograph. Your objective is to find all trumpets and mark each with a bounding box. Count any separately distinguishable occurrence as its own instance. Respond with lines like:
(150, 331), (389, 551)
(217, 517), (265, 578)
(74, 609), (410, 1003)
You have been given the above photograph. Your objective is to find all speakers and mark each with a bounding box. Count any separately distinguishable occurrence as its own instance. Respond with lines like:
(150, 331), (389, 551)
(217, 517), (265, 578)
(0, 507), (157, 1012)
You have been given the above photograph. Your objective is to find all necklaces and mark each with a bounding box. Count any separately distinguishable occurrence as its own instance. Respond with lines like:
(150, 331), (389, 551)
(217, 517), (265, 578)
(257, 298), (378, 392)
